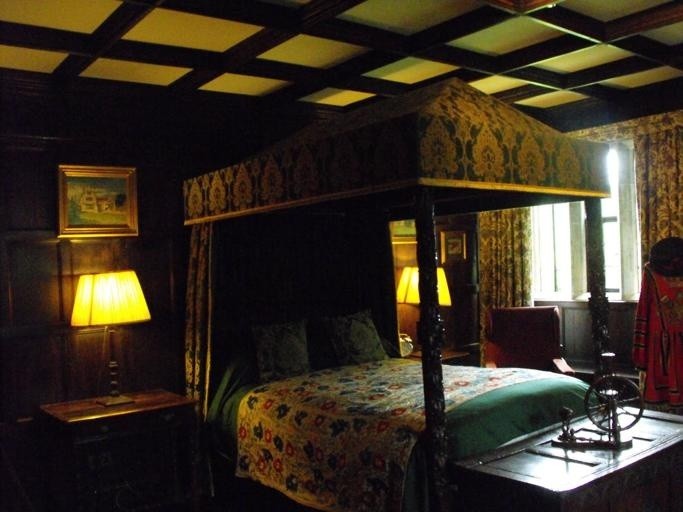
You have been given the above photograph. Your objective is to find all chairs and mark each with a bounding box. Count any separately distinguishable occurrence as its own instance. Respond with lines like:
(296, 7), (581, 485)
(479, 306), (576, 378)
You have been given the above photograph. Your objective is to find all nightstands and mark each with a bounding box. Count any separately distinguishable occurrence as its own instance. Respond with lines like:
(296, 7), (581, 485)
(411, 340), (474, 365)
(35, 387), (199, 512)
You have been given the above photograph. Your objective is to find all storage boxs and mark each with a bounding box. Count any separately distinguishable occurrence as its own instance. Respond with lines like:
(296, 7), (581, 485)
(451, 403), (683, 511)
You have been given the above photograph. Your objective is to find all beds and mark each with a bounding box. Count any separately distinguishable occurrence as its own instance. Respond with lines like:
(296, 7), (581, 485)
(187, 355), (600, 511)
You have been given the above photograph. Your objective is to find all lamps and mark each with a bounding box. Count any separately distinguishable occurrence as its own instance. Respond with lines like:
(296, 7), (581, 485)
(404, 266), (453, 345)
(68, 269), (154, 407)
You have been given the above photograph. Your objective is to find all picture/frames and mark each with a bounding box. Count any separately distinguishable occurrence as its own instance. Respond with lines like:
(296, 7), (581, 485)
(439, 229), (469, 265)
(55, 163), (142, 239)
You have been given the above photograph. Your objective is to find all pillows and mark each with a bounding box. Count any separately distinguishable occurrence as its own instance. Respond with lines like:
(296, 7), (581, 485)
(249, 307), (392, 390)
